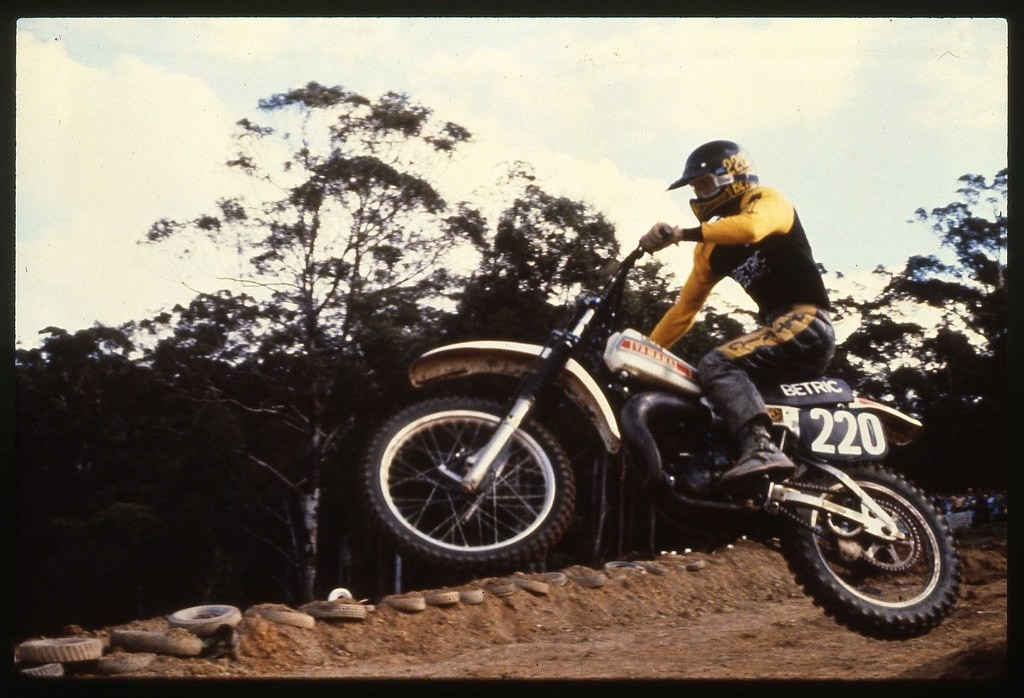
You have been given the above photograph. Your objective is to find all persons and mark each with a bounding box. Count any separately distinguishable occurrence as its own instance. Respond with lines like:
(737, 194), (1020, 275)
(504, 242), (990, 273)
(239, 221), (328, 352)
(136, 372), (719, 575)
(638, 140), (835, 491)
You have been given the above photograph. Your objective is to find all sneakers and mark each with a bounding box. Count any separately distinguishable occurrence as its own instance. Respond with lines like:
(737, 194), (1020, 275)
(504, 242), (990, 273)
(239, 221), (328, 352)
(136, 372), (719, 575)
(722, 424), (797, 489)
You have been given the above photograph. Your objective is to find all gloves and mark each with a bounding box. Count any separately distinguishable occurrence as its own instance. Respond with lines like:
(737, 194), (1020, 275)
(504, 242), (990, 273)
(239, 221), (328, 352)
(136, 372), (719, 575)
(639, 222), (679, 253)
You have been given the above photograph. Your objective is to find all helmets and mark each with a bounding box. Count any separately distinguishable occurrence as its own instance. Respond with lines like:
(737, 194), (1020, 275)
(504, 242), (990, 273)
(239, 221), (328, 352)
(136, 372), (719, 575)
(667, 140), (759, 222)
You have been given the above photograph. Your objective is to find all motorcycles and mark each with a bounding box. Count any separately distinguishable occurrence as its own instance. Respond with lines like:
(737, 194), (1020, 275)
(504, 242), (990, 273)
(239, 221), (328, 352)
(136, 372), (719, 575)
(355, 222), (963, 640)
